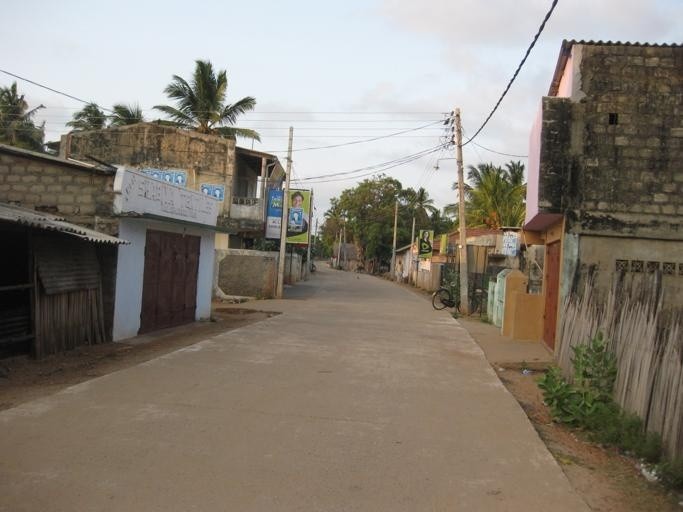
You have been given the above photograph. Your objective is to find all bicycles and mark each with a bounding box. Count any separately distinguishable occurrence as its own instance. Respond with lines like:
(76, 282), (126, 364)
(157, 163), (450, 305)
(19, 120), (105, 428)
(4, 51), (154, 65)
(431, 282), (478, 316)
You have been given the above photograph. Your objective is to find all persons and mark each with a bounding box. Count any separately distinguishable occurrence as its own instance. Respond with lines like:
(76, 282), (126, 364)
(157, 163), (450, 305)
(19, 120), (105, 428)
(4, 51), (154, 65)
(287, 192), (308, 237)
(203, 187), (210, 195)
(292, 211), (300, 221)
(214, 189), (223, 198)
(152, 173), (184, 184)
(420, 231), (432, 255)
